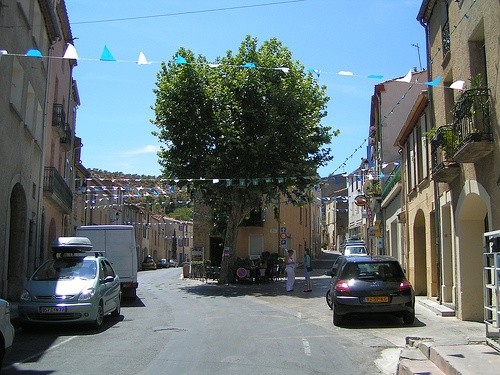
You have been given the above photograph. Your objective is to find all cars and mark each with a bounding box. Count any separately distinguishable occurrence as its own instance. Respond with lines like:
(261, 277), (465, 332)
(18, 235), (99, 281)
(0, 298), (15, 363)
(326, 255), (415, 326)
(138, 258), (179, 272)
(16, 236), (122, 333)
(342, 237), (367, 257)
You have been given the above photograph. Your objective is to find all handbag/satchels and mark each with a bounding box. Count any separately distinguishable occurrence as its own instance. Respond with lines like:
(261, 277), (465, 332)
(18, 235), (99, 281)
(307, 267), (312, 271)
(285, 267), (292, 273)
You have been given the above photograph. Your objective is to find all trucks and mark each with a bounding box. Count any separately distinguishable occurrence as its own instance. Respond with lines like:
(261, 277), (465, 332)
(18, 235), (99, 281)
(76, 224), (139, 302)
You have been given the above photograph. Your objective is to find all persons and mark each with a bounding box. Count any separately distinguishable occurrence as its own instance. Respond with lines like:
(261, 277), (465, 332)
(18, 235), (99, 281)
(303, 248), (313, 293)
(285, 250), (296, 292)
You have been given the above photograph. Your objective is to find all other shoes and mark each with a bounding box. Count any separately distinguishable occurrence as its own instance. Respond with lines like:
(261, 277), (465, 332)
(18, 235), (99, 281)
(304, 289), (312, 292)
(286, 289), (294, 293)
